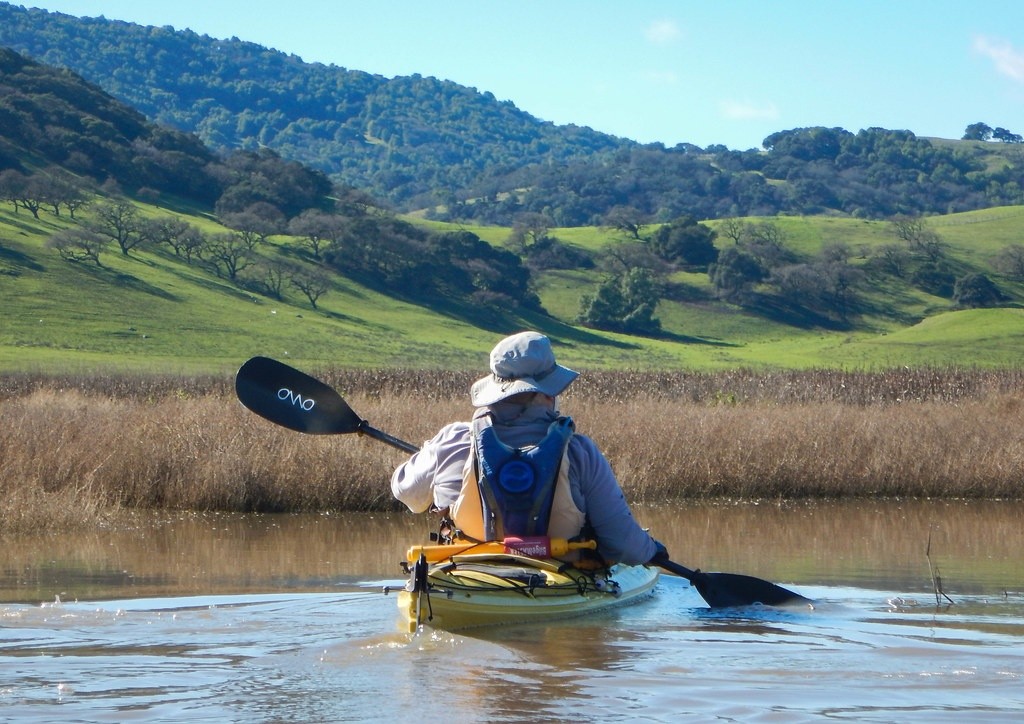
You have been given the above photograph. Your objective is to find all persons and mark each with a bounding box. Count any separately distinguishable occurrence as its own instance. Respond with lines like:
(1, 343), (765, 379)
(390, 331), (670, 567)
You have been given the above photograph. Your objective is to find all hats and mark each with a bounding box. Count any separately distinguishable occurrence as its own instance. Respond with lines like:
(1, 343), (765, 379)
(470, 332), (581, 407)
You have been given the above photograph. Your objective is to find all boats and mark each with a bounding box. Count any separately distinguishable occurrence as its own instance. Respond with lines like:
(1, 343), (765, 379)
(395, 554), (663, 630)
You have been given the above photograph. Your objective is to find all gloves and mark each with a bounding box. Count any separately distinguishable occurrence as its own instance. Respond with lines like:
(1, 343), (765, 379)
(650, 537), (669, 566)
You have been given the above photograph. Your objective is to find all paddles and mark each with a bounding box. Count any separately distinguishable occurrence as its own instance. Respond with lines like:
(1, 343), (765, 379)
(236, 355), (813, 608)
